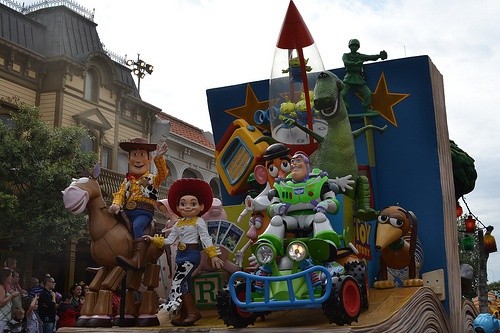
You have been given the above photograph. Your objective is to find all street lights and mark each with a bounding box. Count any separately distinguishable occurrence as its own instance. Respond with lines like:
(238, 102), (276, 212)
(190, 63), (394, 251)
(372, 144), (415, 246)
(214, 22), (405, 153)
(126, 54), (154, 94)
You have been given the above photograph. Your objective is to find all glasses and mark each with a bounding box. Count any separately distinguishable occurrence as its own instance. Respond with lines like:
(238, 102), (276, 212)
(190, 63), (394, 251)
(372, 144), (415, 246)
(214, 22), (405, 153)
(49, 282), (55, 283)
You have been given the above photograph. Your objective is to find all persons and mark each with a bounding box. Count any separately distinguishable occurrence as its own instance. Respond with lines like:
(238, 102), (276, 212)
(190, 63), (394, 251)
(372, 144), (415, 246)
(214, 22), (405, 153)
(237, 197), (281, 269)
(109, 137), (168, 270)
(251, 152), (341, 268)
(0, 257), (124, 333)
(340, 38), (388, 114)
(255, 142), (355, 192)
(142, 178), (225, 326)
